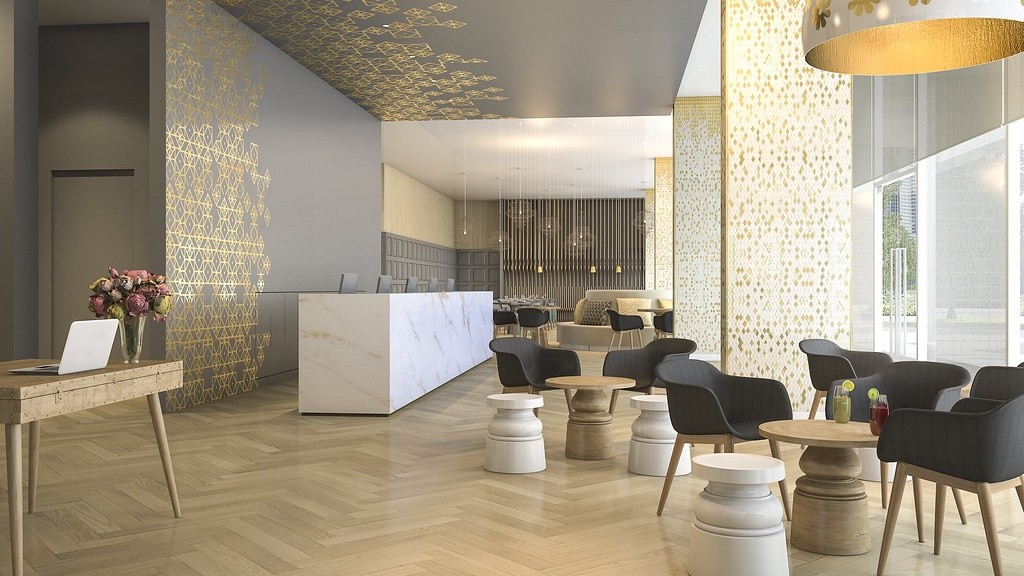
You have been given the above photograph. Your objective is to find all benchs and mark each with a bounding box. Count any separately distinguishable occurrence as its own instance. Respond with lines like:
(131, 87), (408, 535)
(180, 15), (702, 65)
(557, 290), (673, 352)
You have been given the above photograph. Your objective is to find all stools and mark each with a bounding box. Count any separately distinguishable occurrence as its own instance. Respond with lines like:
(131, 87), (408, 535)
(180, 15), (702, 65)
(687, 453), (789, 576)
(483, 393), (546, 474)
(627, 395), (692, 476)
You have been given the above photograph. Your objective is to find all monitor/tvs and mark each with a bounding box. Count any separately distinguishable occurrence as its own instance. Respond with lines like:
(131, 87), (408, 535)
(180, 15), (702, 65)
(340, 273), (359, 294)
(376, 276), (392, 293)
(405, 278), (418, 293)
(445, 279), (454, 292)
(427, 278), (438, 292)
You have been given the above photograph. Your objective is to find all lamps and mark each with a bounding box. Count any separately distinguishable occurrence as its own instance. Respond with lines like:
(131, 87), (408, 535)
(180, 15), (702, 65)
(546, 190), (553, 228)
(577, 184), (585, 238)
(803, 0), (1024, 76)
(516, 167), (525, 215)
(537, 199), (543, 274)
(460, 172), (469, 234)
(616, 197), (622, 274)
(571, 184), (579, 246)
(496, 178), (504, 243)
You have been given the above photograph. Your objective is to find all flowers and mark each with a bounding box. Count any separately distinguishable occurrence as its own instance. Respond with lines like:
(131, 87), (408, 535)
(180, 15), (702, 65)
(89, 267), (171, 358)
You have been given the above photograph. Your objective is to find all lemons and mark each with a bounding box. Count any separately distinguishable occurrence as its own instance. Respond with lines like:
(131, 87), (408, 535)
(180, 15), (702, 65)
(842, 380), (854, 391)
(867, 387), (879, 400)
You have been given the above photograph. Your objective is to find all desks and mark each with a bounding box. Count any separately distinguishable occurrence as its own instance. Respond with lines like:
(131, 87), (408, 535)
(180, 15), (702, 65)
(493, 298), (562, 330)
(759, 419), (880, 557)
(545, 376), (636, 461)
(637, 309), (673, 340)
(0, 358), (184, 576)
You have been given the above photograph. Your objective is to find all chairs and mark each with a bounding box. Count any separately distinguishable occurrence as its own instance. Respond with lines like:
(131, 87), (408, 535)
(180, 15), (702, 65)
(799, 339), (1024, 576)
(654, 311), (673, 339)
(606, 310), (644, 353)
(516, 308), (549, 347)
(493, 308), (518, 340)
(489, 337), (581, 418)
(603, 338), (697, 447)
(655, 359), (793, 521)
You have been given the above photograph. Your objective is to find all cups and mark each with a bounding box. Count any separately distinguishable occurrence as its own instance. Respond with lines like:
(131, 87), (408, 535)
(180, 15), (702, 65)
(833, 386), (851, 424)
(869, 394), (889, 436)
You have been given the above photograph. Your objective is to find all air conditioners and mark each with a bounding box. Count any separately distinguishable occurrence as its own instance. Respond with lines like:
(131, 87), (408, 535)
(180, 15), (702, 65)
(591, 198), (596, 274)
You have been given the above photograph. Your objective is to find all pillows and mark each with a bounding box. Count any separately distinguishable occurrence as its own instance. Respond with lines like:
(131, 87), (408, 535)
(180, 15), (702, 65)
(580, 300), (612, 326)
(657, 299), (673, 309)
(574, 299), (588, 324)
(616, 298), (652, 326)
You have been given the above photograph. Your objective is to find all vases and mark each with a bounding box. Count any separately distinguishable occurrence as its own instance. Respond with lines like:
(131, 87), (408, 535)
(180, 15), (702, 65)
(117, 316), (146, 364)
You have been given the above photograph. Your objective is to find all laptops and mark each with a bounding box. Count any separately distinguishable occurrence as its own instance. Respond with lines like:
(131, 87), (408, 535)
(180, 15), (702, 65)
(8, 319), (119, 375)
